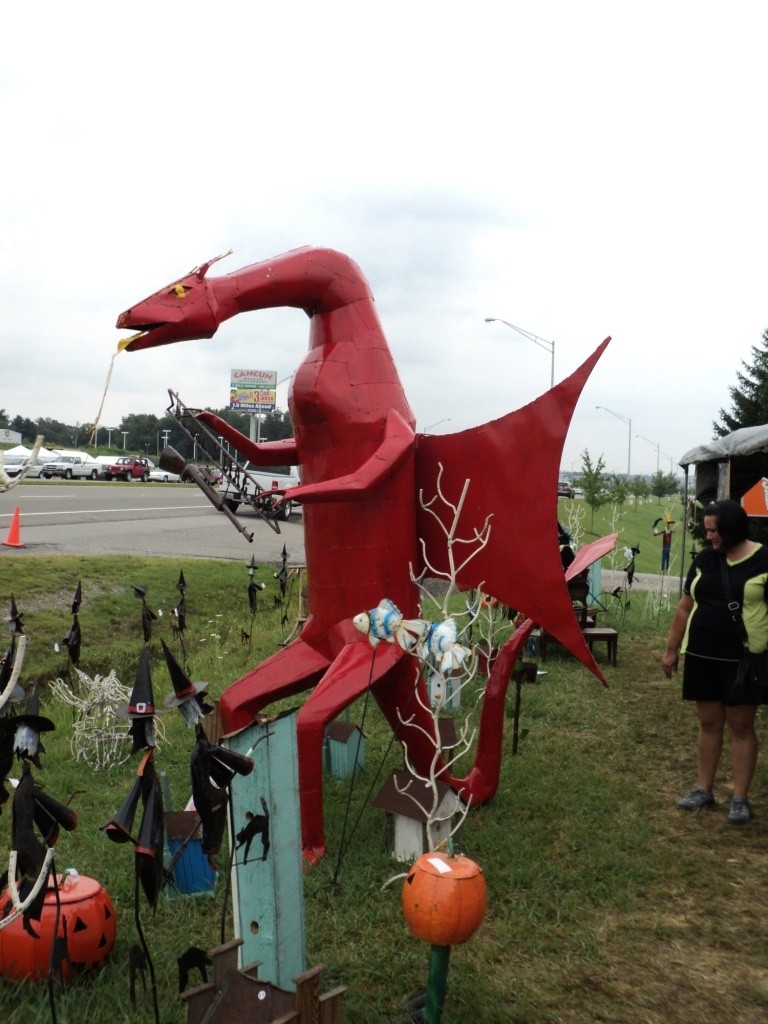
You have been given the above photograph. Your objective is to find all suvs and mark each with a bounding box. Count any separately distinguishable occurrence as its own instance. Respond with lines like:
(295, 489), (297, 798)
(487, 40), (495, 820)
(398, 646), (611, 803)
(104, 457), (150, 481)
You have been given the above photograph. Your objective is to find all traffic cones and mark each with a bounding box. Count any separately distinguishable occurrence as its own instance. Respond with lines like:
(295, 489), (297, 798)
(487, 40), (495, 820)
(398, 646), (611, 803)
(0, 507), (27, 547)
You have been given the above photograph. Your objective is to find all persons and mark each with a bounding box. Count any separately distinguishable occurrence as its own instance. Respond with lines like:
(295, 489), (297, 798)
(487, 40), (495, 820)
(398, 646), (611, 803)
(653, 513), (675, 571)
(662, 499), (768, 821)
(210, 468), (216, 486)
(204, 465), (211, 485)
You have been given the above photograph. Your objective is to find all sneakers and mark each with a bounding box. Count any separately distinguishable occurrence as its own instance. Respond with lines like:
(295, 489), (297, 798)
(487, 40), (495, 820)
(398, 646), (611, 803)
(675, 783), (716, 812)
(726, 796), (755, 825)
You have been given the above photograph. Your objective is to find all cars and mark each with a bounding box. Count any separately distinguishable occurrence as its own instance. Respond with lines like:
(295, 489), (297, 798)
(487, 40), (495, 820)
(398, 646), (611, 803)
(148, 466), (182, 483)
(556, 481), (576, 499)
(3, 456), (46, 480)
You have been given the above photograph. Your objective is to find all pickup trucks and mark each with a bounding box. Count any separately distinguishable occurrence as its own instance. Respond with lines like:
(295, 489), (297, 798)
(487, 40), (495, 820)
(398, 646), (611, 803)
(217, 461), (303, 522)
(42, 456), (104, 481)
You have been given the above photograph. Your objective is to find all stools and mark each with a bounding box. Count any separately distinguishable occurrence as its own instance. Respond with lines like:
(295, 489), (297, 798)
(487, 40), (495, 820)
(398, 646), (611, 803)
(583, 625), (618, 667)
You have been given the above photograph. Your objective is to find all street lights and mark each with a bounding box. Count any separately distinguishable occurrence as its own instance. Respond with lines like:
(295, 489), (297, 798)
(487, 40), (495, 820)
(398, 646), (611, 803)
(106, 428), (115, 448)
(121, 431), (129, 453)
(483, 316), (555, 390)
(225, 441), (231, 465)
(161, 429), (172, 449)
(193, 432), (200, 459)
(635, 432), (660, 481)
(217, 436), (224, 465)
(596, 404), (633, 485)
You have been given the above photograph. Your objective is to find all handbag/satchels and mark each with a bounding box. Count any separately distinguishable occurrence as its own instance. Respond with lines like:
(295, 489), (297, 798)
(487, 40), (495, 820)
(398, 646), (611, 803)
(724, 649), (768, 706)
